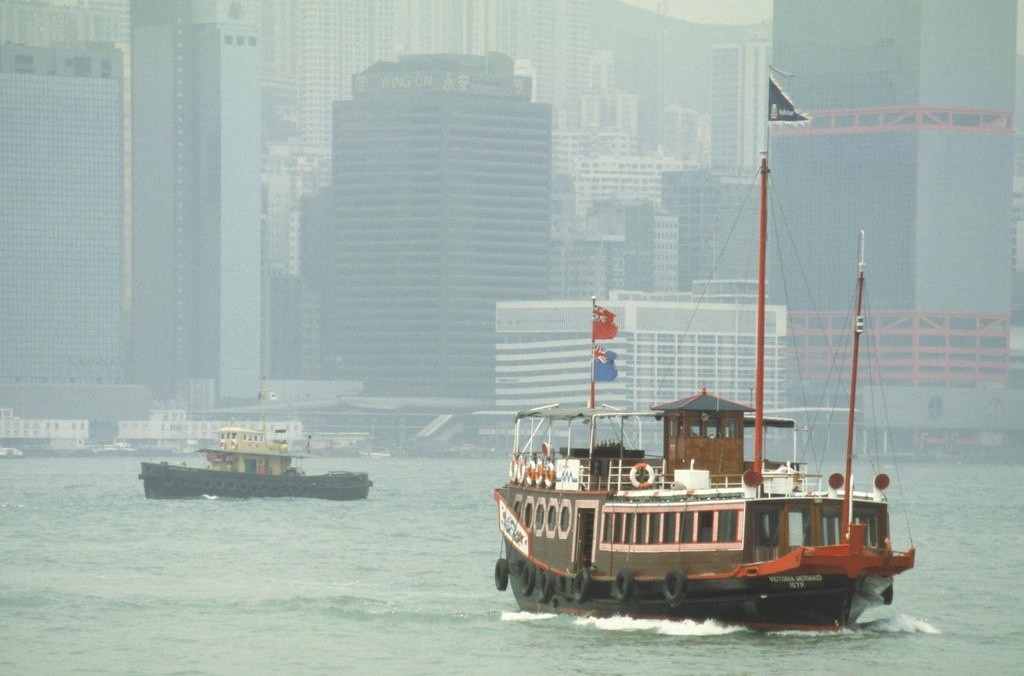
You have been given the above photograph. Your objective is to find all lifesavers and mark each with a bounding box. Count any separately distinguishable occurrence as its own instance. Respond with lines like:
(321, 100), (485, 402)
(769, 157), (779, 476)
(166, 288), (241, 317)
(526, 460), (536, 486)
(535, 461), (544, 485)
(516, 458), (526, 482)
(544, 462), (555, 488)
(219, 440), (226, 450)
(509, 459), (516, 483)
(231, 440), (238, 450)
(629, 462), (655, 489)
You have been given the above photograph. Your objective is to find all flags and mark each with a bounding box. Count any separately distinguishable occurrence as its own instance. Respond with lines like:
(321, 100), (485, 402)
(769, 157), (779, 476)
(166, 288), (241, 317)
(592, 305), (617, 340)
(591, 345), (618, 382)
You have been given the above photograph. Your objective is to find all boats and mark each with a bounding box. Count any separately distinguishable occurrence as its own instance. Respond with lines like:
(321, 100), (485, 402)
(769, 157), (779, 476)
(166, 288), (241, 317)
(0, 447), (22, 459)
(181, 439), (202, 456)
(492, 65), (917, 634)
(92, 441), (136, 453)
(136, 377), (372, 505)
(360, 446), (390, 460)
